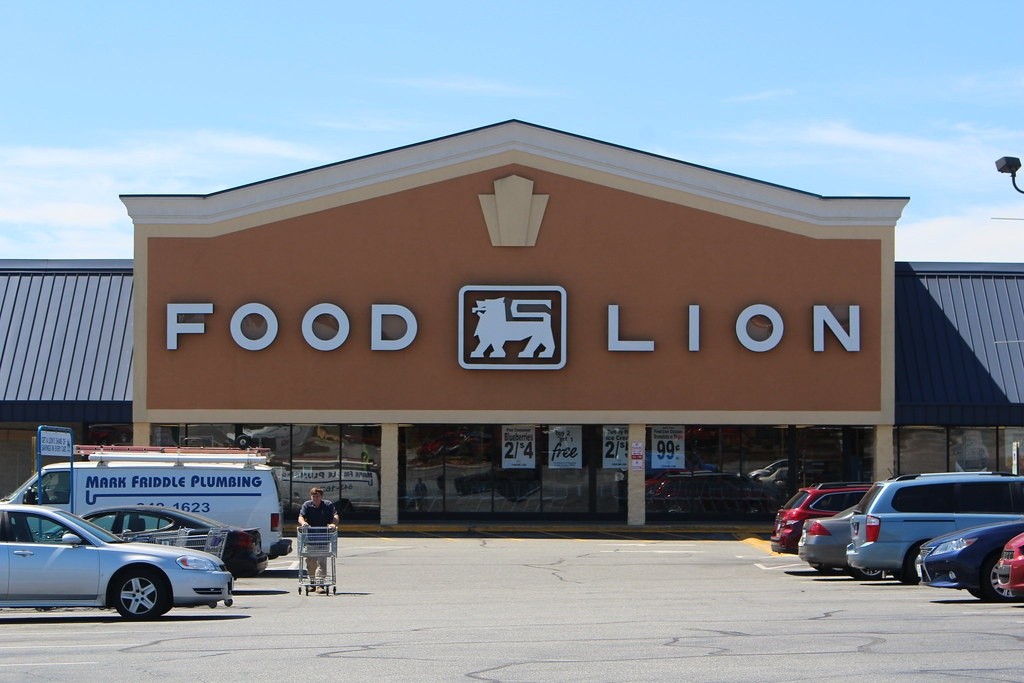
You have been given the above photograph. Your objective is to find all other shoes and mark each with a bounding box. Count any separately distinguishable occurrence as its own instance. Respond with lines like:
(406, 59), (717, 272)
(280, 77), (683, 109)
(307, 586), (315, 592)
(316, 586), (326, 594)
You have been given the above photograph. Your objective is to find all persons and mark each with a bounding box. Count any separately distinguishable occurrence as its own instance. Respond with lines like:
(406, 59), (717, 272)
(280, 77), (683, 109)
(298, 487), (339, 593)
(414, 478), (427, 503)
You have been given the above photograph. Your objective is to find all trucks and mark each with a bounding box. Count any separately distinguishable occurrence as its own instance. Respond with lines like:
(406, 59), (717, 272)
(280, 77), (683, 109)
(272, 459), (381, 522)
(0, 451), (292, 562)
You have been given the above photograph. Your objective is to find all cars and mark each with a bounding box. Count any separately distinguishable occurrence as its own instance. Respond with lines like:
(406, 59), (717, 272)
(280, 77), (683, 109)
(798, 504), (891, 581)
(645, 459), (874, 519)
(1, 502), (233, 620)
(996, 531), (1024, 590)
(80, 505), (268, 579)
(916, 522), (1023, 601)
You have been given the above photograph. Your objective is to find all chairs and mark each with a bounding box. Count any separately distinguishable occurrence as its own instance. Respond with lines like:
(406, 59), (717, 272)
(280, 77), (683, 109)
(131, 518), (148, 532)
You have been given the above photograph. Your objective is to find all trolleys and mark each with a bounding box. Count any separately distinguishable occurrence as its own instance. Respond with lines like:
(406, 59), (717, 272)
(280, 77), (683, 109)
(111, 525), (229, 563)
(296, 526), (339, 596)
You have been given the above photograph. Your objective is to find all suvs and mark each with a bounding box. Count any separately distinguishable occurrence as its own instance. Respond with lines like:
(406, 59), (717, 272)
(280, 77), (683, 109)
(770, 480), (875, 554)
(847, 470), (1023, 583)
